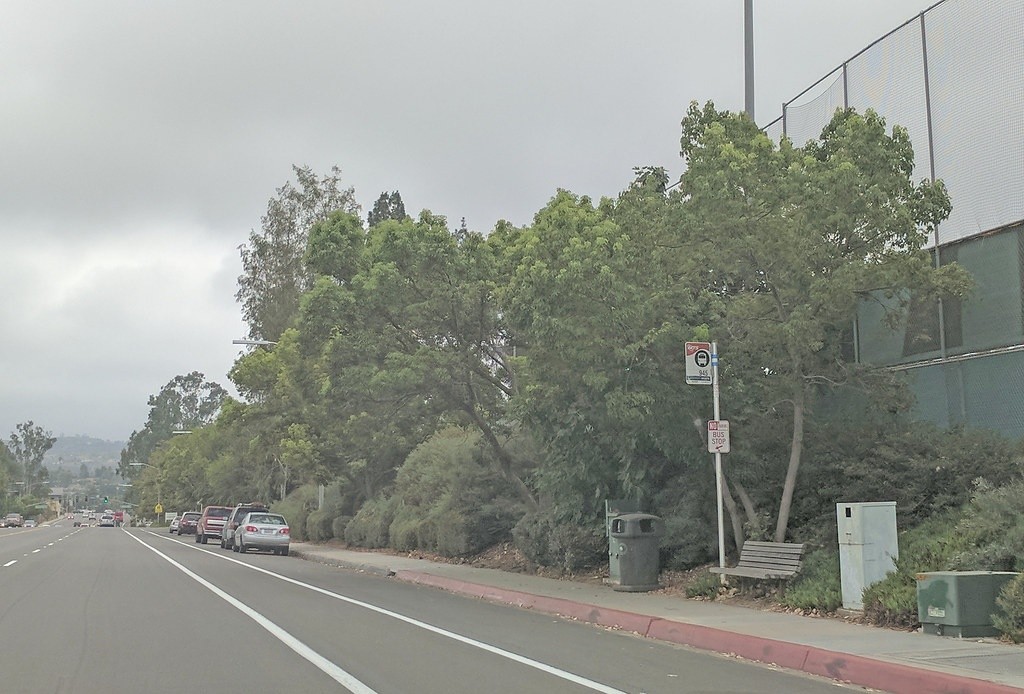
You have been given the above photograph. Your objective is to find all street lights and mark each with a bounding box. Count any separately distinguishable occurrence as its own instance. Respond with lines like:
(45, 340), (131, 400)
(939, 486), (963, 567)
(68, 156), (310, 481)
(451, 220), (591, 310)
(232, 339), (326, 507)
(127, 462), (161, 526)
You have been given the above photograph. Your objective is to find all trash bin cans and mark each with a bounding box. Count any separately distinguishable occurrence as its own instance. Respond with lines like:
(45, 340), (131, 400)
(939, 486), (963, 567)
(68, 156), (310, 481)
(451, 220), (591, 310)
(609, 513), (666, 591)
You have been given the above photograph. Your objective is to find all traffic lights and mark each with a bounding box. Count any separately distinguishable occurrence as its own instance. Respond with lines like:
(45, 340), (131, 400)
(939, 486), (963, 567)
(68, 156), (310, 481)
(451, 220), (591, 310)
(104, 497), (108, 503)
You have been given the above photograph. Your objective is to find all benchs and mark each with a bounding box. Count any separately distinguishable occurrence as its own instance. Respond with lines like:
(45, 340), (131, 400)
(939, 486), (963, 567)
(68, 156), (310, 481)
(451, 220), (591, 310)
(711, 540), (810, 599)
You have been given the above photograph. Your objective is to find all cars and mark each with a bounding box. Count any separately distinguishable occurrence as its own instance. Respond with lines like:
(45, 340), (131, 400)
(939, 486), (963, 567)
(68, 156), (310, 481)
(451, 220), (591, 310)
(73, 509), (124, 527)
(0, 519), (5, 528)
(23, 519), (35, 528)
(170, 515), (182, 533)
(68, 514), (74, 519)
(196, 506), (236, 544)
(233, 512), (291, 556)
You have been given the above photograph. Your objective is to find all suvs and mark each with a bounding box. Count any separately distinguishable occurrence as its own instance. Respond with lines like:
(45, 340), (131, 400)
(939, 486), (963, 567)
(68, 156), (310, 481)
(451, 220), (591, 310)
(3, 513), (24, 528)
(222, 503), (271, 550)
(177, 511), (203, 536)
